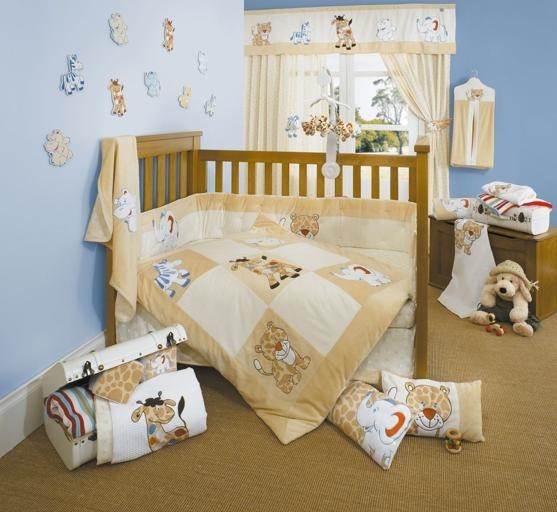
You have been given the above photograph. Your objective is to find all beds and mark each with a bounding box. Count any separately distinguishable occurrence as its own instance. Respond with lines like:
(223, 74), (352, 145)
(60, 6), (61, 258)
(108, 133), (432, 388)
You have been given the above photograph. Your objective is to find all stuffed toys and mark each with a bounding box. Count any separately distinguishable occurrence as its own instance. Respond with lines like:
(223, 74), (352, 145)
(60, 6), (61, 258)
(302, 94), (360, 142)
(485, 320), (507, 336)
(470, 259), (541, 336)
(444, 427), (462, 453)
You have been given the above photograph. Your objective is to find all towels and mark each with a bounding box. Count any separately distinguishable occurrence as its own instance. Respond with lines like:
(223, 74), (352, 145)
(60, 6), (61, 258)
(84, 134), (140, 324)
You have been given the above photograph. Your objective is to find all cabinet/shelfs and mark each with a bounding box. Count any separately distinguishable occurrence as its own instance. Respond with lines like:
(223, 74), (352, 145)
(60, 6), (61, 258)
(428, 213), (557, 319)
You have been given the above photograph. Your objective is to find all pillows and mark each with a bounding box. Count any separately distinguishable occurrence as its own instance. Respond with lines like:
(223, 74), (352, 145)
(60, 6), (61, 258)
(328, 370), (485, 472)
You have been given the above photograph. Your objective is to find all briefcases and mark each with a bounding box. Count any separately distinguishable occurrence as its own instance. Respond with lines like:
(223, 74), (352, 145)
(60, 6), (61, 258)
(474, 199), (550, 236)
